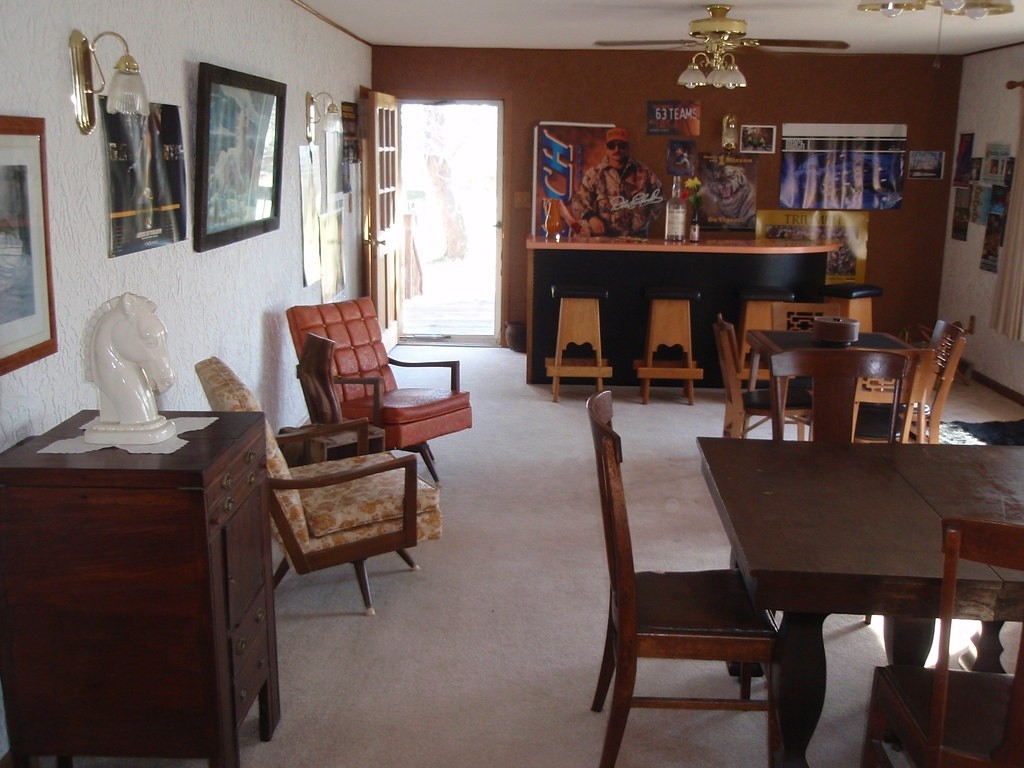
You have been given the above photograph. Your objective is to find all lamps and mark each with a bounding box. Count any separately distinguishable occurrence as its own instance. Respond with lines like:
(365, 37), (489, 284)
(853, 0), (1014, 21)
(677, 42), (746, 89)
(67, 26), (150, 135)
(721, 112), (739, 151)
(305, 91), (344, 142)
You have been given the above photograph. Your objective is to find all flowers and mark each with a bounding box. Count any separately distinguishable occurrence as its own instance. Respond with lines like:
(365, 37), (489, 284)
(679, 177), (705, 209)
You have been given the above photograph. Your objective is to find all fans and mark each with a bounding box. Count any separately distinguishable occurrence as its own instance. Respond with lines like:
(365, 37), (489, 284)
(593, 4), (849, 58)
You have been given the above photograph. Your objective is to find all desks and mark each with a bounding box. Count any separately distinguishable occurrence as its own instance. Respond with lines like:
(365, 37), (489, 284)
(695, 436), (1024, 768)
(278, 415), (387, 463)
(525, 234), (841, 256)
(744, 329), (919, 438)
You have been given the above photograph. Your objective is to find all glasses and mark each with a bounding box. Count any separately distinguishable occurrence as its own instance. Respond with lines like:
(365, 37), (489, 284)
(607, 142), (629, 150)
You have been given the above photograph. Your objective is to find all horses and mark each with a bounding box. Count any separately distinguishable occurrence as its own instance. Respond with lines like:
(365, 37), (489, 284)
(78, 290), (175, 429)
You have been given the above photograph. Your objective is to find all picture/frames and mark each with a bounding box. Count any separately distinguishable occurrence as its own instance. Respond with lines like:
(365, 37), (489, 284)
(0, 115), (59, 377)
(739, 124), (777, 154)
(192, 61), (287, 253)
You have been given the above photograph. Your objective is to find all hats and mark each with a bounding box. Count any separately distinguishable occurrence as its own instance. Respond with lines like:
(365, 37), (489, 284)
(607, 129), (630, 144)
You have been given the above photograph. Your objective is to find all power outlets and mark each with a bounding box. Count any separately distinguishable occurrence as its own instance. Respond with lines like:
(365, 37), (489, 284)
(969, 315), (976, 335)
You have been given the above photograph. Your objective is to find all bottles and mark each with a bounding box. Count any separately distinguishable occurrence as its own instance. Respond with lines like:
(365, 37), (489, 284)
(689, 208), (699, 242)
(664, 175), (686, 243)
(544, 199), (561, 238)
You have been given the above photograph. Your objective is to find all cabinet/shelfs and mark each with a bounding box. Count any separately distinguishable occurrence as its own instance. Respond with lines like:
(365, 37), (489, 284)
(0, 409), (281, 768)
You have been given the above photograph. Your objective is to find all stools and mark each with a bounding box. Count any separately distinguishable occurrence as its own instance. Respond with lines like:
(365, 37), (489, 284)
(736, 284), (797, 381)
(631, 285), (705, 405)
(544, 282), (612, 403)
(817, 282), (883, 334)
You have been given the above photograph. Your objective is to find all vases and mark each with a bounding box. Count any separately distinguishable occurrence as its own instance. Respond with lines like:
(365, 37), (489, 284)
(504, 320), (527, 354)
(688, 206), (702, 242)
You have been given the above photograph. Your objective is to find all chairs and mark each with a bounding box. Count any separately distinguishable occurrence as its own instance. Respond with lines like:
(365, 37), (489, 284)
(194, 356), (442, 616)
(286, 295), (474, 488)
(584, 386), (780, 768)
(860, 514), (1024, 768)
(711, 311), (967, 444)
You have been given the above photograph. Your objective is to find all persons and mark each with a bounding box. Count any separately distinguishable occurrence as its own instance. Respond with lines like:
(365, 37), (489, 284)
(573, 128), (666, 238)
